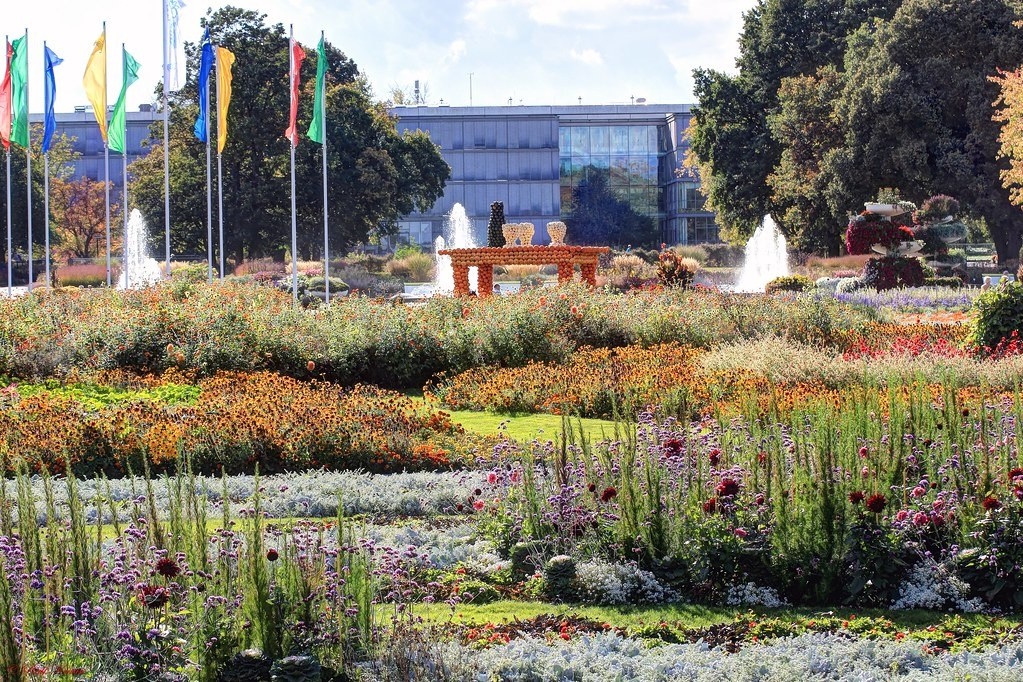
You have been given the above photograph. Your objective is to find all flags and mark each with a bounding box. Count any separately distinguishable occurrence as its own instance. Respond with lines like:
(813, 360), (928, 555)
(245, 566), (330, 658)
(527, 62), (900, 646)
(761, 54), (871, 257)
(107, 48), (141, 153)
(83, 32), (107, 140)
(0, 40), (18, 151)
(10, 34), (28, 147)
(194, 27), (215, 142)
(285, 38), (306, 148)
(40, 45), (64, 154)
(215, 44), (235, 154)
(166, 0), (187, 91)
(307, 36), (329, 144)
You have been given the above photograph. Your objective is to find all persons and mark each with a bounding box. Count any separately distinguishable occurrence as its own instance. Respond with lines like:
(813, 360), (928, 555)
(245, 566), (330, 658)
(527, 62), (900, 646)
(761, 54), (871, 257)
(1002, 270), (1009, 279)
(626, 245), (631, 252)
(981, 276), (993, 292)
(493, 283), (502, 295)
